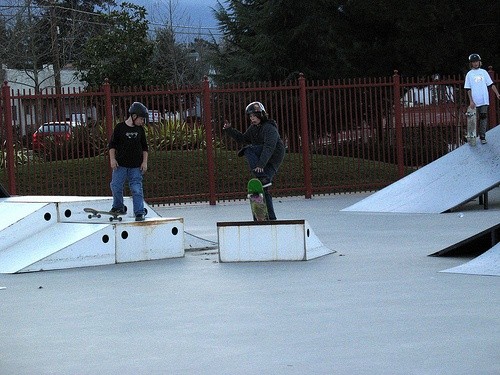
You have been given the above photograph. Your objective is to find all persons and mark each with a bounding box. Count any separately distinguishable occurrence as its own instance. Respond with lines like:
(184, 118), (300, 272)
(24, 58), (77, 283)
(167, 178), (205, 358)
(463, 54), (500, 144)
(223, 102), (285, 219)
(109, 102), (149, 221)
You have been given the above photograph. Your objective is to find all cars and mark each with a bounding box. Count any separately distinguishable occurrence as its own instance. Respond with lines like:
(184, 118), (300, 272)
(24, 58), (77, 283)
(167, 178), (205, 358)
(0, 119), (31, 150)
(147, 109), (159, 123)
(33, 121), (81, 153)
(161, 109), (189, 120)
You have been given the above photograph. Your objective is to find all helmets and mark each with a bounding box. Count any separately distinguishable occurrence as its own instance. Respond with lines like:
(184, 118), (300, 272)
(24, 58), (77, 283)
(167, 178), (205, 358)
(129, 102), (148, 117)
(245, 102), (265, 113)
(470, 54), (481, 61)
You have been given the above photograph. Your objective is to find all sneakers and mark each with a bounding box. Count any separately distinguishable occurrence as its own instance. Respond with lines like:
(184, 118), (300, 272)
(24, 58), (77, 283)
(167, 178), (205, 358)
(260, 177), (272, 187)
(136, 213), (145, 220)
(110, 206), (127, 214)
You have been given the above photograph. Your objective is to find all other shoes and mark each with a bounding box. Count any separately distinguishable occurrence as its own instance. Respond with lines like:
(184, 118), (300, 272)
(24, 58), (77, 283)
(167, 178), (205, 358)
(482, 137), (488, 143)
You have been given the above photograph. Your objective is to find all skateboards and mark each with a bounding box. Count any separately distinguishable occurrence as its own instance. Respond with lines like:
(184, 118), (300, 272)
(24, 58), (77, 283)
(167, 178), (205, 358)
(465, 106), (476, 146)
(84, 208), (126, 221)
(248, 178), (269, 221)
